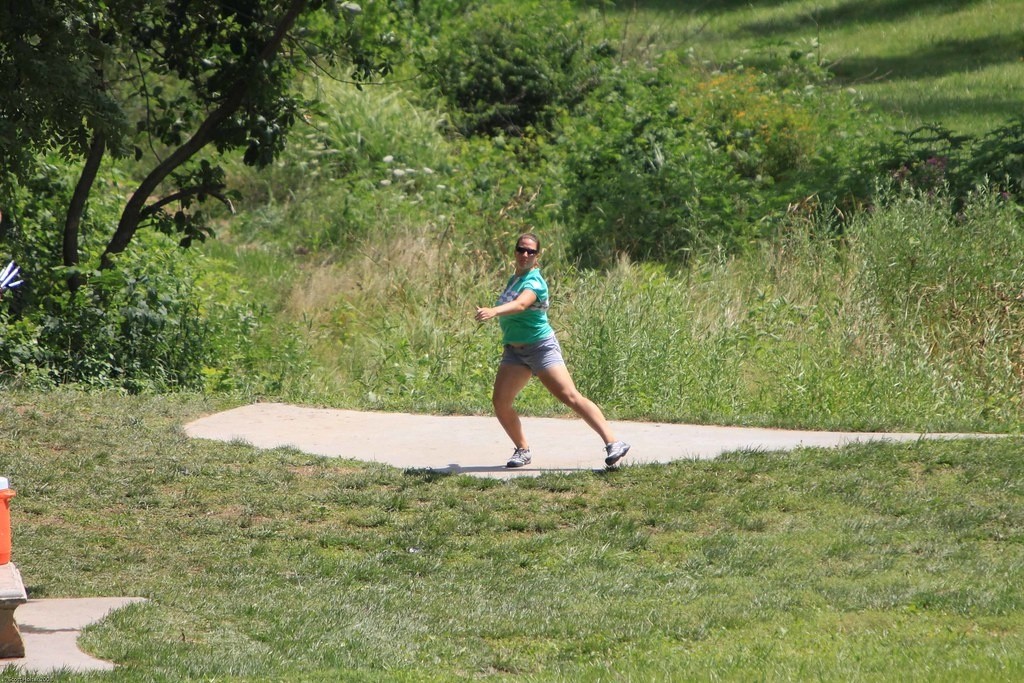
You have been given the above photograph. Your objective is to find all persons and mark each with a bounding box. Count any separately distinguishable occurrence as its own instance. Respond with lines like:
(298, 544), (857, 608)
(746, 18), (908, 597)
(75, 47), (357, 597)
(474, 233), (630, 468)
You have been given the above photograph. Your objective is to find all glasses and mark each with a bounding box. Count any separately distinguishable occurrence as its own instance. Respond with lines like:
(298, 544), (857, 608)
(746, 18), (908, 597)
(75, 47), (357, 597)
(516, 246), (538, 254)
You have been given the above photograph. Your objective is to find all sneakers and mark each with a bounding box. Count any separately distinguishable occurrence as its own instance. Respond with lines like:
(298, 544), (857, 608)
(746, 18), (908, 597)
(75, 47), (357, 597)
(605, 440), (630, 466)
(507, 447), (530, 467)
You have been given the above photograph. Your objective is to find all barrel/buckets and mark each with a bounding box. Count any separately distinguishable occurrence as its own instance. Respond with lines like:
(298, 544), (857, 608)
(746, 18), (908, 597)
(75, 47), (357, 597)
(0, 477), (11, 565)
